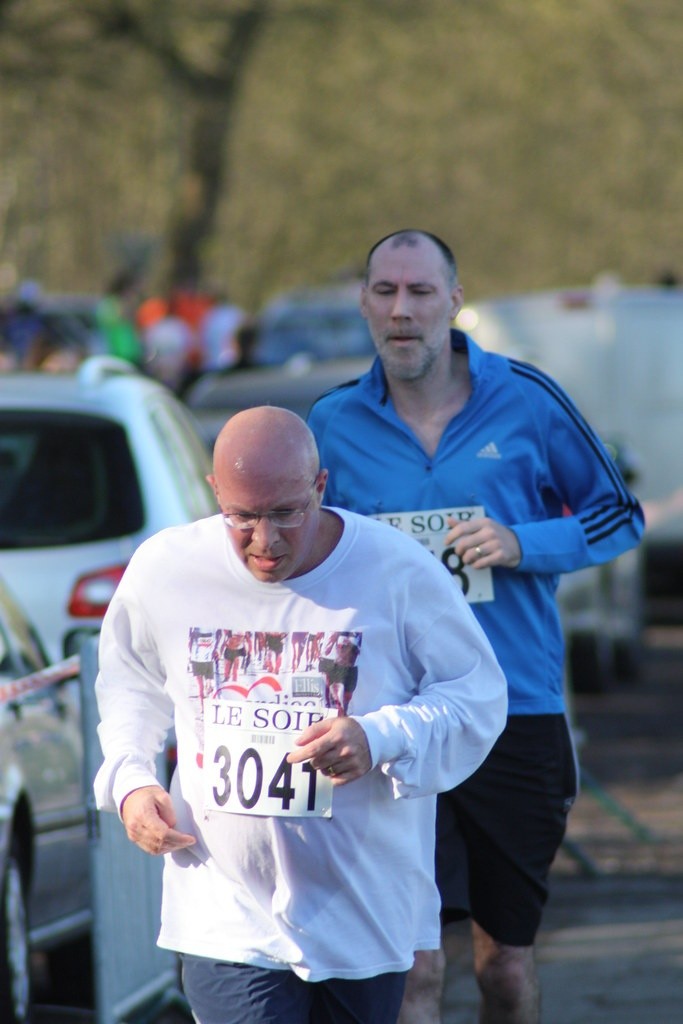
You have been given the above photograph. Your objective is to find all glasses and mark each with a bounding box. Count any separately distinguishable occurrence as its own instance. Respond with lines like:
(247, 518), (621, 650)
(221, 473), (324, 530)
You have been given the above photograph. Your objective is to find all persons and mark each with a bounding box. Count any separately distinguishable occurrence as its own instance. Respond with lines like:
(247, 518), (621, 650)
(2, 271), (251, 401)
(305, 229), (644, 1024)
(94, 406), (509, 1024)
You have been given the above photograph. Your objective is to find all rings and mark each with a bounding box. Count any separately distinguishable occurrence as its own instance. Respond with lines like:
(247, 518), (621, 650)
(328, 766), (336, 779)
(475, 548), (484, 557)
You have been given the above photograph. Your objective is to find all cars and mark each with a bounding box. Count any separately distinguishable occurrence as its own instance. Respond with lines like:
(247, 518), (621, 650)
(0, 578), (100, 1024)
(178, 286), (683, 691)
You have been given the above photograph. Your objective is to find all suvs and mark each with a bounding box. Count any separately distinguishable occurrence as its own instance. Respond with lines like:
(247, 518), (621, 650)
(0, 351), (227, 802)
